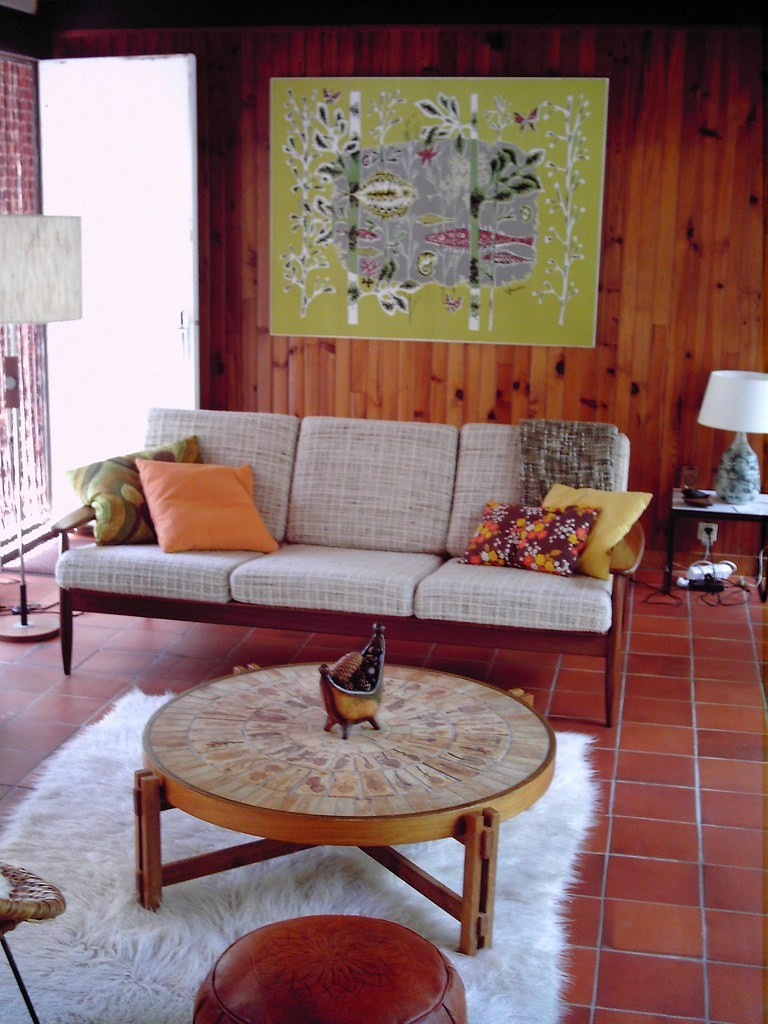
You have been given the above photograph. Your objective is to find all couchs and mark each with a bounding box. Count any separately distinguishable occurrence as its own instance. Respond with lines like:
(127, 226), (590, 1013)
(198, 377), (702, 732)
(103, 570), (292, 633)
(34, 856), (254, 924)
(55, 409), (646, 728)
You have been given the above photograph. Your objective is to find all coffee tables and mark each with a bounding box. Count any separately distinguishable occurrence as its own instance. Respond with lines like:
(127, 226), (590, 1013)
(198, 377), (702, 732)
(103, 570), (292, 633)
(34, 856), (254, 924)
(133, 661), (557, 956)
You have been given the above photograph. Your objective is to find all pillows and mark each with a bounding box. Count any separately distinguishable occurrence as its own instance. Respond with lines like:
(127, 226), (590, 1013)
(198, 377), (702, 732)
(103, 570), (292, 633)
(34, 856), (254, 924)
(67, 435), (204, 547)
(134, 458), (280, 554)
(458, 500), (601, 577)
(541, 484), (654, 580)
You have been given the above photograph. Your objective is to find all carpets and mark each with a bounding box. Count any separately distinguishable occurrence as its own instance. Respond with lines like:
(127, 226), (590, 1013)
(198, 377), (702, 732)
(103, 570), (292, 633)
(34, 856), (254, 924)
(1, 688), (601, 1024)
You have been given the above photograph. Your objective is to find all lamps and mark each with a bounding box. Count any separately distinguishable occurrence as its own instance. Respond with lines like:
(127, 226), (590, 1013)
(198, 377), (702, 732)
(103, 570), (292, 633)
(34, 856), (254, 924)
(0, 214), (82, 641)
(698, 370), (768, 505)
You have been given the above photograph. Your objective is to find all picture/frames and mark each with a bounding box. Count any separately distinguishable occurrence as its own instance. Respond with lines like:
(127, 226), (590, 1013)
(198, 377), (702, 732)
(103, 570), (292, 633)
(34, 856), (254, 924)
(270, 77), (609, 348)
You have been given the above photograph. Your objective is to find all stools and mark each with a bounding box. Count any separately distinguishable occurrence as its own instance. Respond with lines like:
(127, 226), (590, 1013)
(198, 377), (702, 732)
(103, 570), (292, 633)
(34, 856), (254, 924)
(193, 915), (467, 1024)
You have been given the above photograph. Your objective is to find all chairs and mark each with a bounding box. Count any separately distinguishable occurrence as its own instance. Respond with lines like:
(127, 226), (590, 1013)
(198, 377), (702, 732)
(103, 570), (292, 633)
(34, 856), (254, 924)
(0, 861), (67, 1024)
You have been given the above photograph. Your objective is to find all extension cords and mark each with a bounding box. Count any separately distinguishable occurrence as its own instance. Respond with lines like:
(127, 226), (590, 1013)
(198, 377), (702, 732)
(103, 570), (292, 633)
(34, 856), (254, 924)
(689, 578), (724, 592)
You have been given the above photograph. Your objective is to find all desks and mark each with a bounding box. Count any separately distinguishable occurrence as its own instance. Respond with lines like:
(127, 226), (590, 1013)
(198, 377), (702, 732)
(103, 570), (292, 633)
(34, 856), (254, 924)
(663, 488), (768, 601)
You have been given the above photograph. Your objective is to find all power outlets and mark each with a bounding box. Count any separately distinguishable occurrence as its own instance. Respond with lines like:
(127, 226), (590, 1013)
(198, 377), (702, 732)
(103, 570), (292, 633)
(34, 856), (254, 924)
(698, 522), (718, 541)
